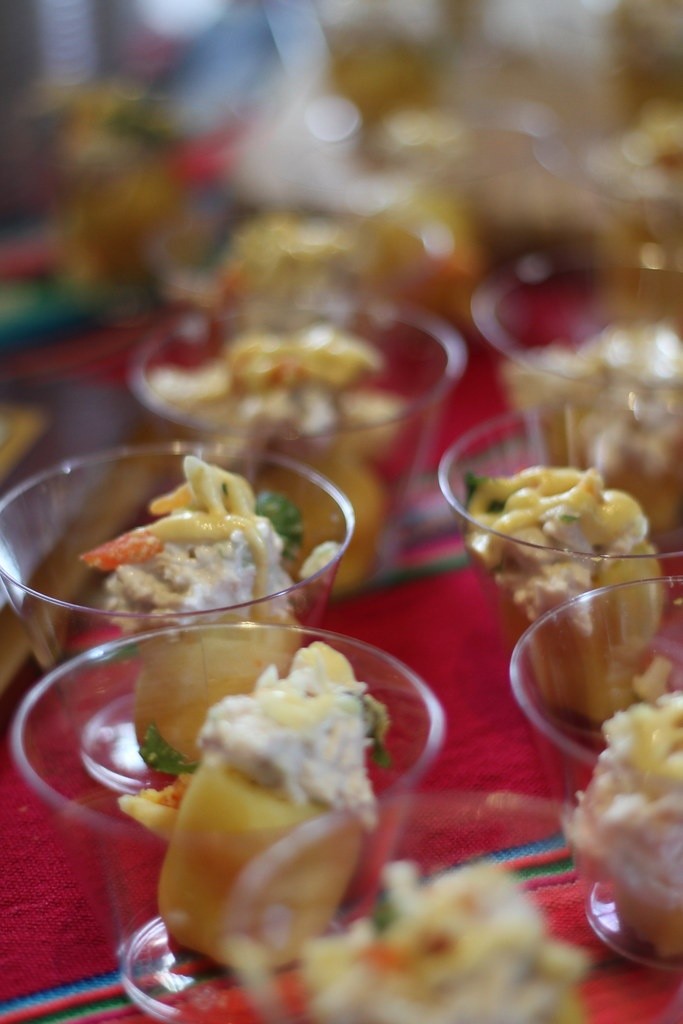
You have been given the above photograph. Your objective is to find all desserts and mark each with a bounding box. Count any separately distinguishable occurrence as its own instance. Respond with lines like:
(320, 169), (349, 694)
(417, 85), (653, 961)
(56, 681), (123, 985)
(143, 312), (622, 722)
(81, 169), (683, 1024)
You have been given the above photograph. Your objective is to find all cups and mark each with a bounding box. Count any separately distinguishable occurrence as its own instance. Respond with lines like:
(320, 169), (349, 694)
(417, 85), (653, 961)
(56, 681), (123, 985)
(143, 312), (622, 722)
(1, 243), (683, 1024)
